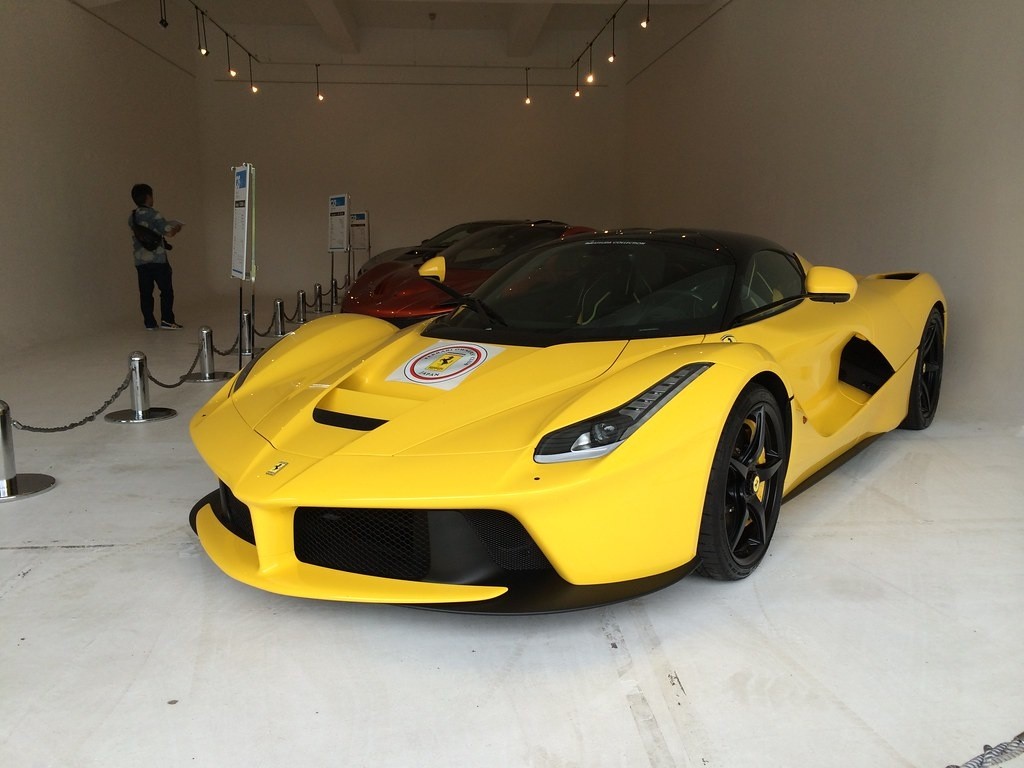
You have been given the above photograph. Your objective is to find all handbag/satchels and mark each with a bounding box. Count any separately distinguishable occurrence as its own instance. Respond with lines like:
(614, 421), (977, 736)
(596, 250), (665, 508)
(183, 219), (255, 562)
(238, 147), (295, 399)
(131, 208), (161, 250)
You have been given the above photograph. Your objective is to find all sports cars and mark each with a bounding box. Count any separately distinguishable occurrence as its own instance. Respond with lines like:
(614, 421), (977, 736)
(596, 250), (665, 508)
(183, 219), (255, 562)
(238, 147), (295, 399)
(188, 229), (949, 615)
(341, 221), (594, 328)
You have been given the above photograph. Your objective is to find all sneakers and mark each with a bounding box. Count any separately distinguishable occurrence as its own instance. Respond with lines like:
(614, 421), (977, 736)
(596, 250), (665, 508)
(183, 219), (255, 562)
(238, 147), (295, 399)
(146, 325), (159, 331)
(161, 320), (183, 330)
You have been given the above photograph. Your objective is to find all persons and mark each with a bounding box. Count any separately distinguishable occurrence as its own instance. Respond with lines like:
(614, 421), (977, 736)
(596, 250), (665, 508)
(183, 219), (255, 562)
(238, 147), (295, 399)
(129, 183), (183, 330)
